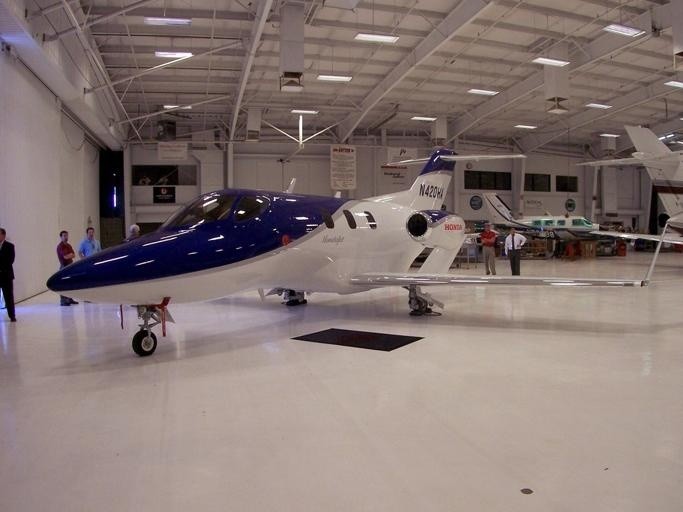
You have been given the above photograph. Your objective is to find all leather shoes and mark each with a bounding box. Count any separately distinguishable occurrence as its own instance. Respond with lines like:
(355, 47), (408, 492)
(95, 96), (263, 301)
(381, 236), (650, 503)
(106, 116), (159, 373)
(60, 299), (78, 306)
(10, 317), (16, 321)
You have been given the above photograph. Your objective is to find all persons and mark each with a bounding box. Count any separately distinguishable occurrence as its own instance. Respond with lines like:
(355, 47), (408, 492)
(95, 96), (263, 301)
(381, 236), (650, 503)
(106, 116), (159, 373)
(123, 224), (140, 241)
(76, 226), (101, 303)
(0, 228), (17, 323)
(502, 227), (528, 276)
(478, 222), (497, 275)
(55, 230), (79, 306)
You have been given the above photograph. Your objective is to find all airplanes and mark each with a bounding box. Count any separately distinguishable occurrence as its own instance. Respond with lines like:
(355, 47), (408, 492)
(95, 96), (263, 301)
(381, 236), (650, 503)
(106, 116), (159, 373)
(473, 191), (625, 258)
(576, 126), (683, 249)
(45, 146), (651, 357)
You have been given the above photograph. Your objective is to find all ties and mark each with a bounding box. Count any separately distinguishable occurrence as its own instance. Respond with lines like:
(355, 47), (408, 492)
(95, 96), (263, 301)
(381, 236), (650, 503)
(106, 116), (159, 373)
(511, 234), (515, 250)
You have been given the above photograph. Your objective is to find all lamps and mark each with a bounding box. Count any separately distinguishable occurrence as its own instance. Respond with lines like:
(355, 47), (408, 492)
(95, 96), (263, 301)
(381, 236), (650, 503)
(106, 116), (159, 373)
(138, 0), (401, 114)
(411, 1), (681, 140)
